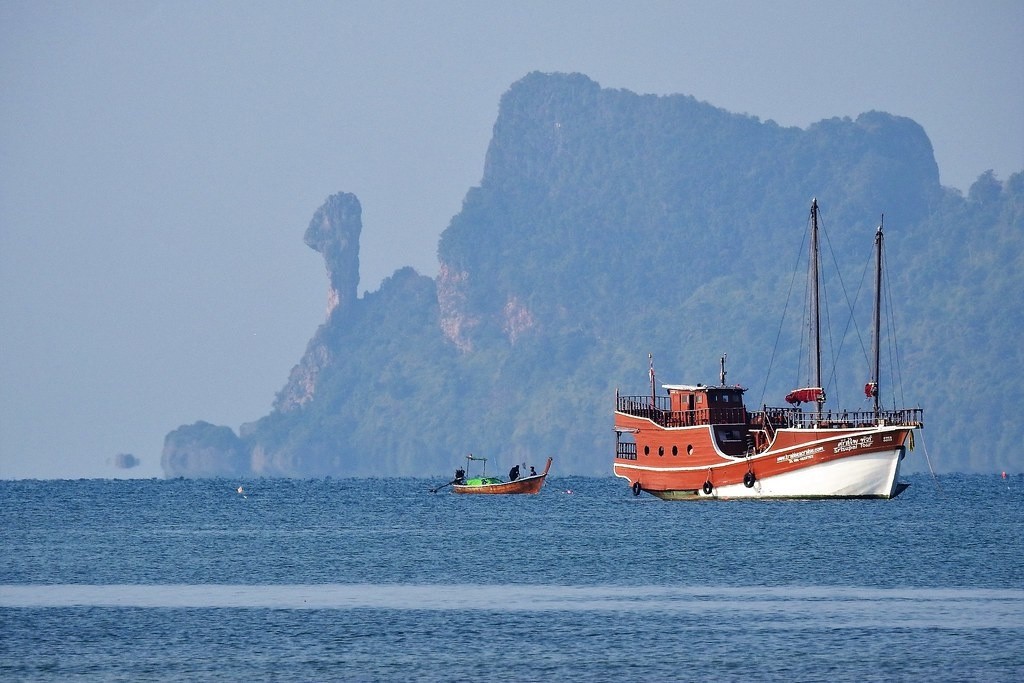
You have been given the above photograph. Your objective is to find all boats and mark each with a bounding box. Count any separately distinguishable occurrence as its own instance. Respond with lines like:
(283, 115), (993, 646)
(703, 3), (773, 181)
(613, 196), (924, 497)
(453, 455), (554, 493)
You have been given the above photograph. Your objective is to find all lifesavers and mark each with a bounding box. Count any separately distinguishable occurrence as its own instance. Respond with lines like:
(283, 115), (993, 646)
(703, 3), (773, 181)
(744, 472), (755, 488)
(703, 481), (712, 494)
(633, 482), (641, 495)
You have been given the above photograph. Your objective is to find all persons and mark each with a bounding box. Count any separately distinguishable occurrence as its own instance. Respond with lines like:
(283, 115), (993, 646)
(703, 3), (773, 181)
(454, 469), (465, 485)
(529, 466), (537, 476)
(509, 465), (520, 481)
